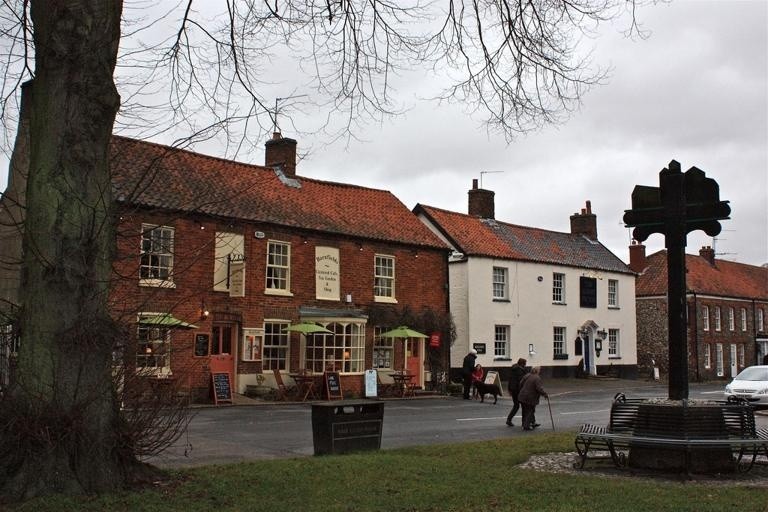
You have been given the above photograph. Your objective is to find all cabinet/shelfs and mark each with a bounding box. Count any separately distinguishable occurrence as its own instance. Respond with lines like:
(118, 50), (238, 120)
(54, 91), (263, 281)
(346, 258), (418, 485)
(239, 327), (266, 361)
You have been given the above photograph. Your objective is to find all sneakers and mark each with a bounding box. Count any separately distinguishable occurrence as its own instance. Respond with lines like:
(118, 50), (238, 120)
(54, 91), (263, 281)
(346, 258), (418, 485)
(502, 419), (542, 433)
(462, 394), (474, 402)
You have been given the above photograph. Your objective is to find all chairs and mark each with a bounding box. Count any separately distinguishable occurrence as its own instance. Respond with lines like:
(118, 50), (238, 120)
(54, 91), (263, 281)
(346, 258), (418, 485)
(272, 366), (325, 402)
(715, 392), (768, 473)
(572, 391), (645, 472)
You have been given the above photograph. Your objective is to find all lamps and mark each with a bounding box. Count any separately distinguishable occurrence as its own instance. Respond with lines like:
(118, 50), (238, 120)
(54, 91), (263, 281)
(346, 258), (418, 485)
(200, 296), (209, 320)
(597, 328), (608, 341)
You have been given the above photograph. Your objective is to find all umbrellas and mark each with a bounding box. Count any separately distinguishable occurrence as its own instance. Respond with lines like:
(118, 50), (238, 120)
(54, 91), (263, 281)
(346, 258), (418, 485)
(277, 319), (336, 374)
(375, 325), (431, 379)
(129, 310), (200, 366)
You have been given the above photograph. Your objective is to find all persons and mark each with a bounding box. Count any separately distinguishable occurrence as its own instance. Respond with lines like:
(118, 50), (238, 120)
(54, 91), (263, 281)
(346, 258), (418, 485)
(461, 348), (478, 401)
(471, 364), (484, 399)
(517, 365), (548, 433)
(504, 358), (541, 429)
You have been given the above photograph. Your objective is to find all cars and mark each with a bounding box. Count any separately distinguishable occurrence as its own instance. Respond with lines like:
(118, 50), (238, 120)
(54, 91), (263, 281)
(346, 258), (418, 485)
(719, 364), (767, 410)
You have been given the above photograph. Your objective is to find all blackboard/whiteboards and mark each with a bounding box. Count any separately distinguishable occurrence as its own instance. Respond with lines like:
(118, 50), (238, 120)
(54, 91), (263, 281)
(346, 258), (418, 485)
(213, 371), (233, 401)
(325, 371), (342, 397)
